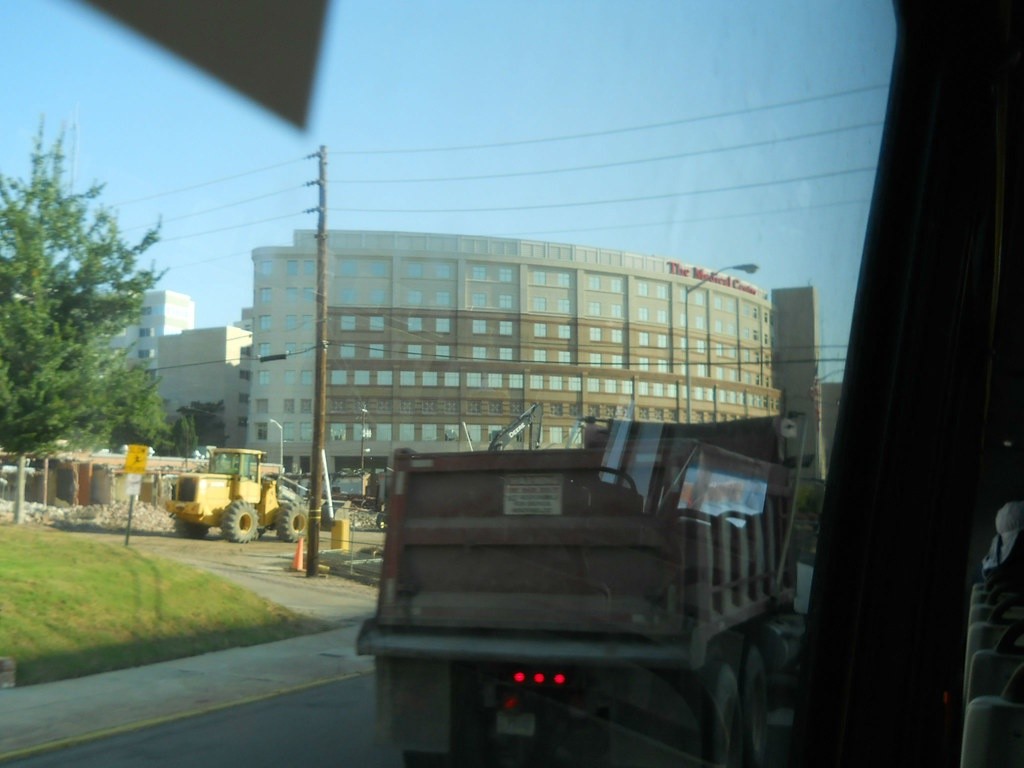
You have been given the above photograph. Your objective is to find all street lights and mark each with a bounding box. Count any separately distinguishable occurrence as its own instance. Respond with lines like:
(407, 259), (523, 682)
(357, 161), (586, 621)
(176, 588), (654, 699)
(686, 263), (763, 424)
(269, 419), (284, 466)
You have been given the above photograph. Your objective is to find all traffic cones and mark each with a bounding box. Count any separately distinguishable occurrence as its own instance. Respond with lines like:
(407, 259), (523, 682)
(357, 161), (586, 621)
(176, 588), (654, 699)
(284, 536), (307, 572)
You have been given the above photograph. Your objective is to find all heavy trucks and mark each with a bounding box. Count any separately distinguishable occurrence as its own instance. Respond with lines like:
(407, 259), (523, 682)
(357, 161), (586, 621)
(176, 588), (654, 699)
(353, 408), (811, 768)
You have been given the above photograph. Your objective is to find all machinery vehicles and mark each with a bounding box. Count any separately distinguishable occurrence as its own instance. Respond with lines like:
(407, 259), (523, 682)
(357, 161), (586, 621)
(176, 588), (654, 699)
(166, 447), (351, 545)
